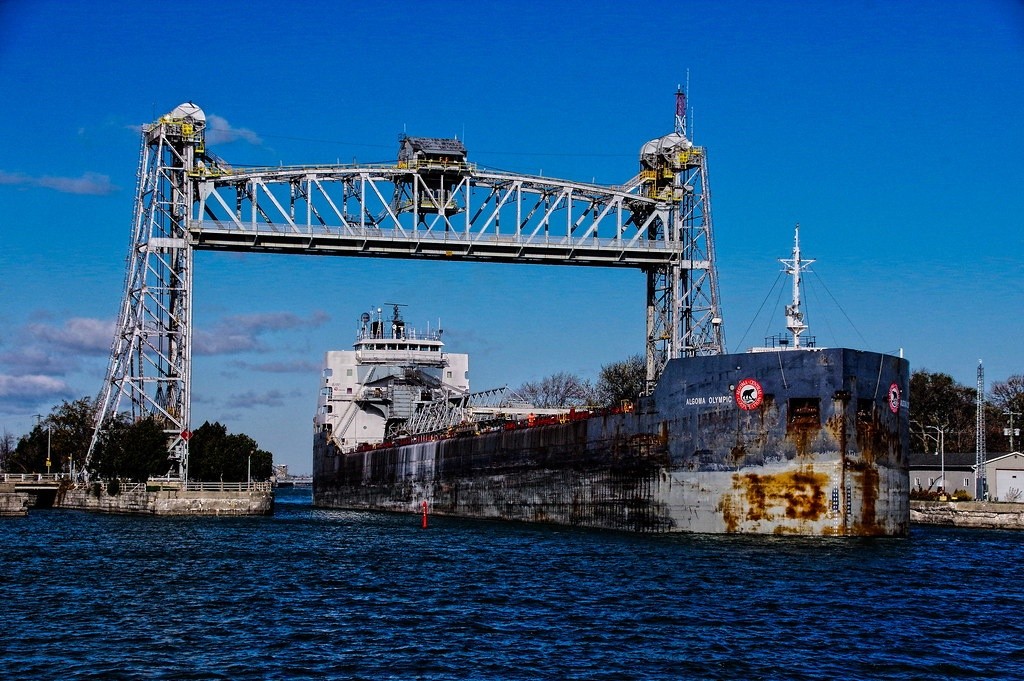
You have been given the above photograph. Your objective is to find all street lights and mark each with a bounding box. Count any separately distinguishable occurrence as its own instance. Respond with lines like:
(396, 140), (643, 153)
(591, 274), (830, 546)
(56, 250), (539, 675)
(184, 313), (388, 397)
(923, 431), (940, 455)
(925, 425), (945, 496)
(248, 450), (254, 492)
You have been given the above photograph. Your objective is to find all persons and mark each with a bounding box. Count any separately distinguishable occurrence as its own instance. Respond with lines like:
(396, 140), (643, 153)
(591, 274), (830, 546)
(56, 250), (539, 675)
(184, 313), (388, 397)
(527, 413), (536, 429)
